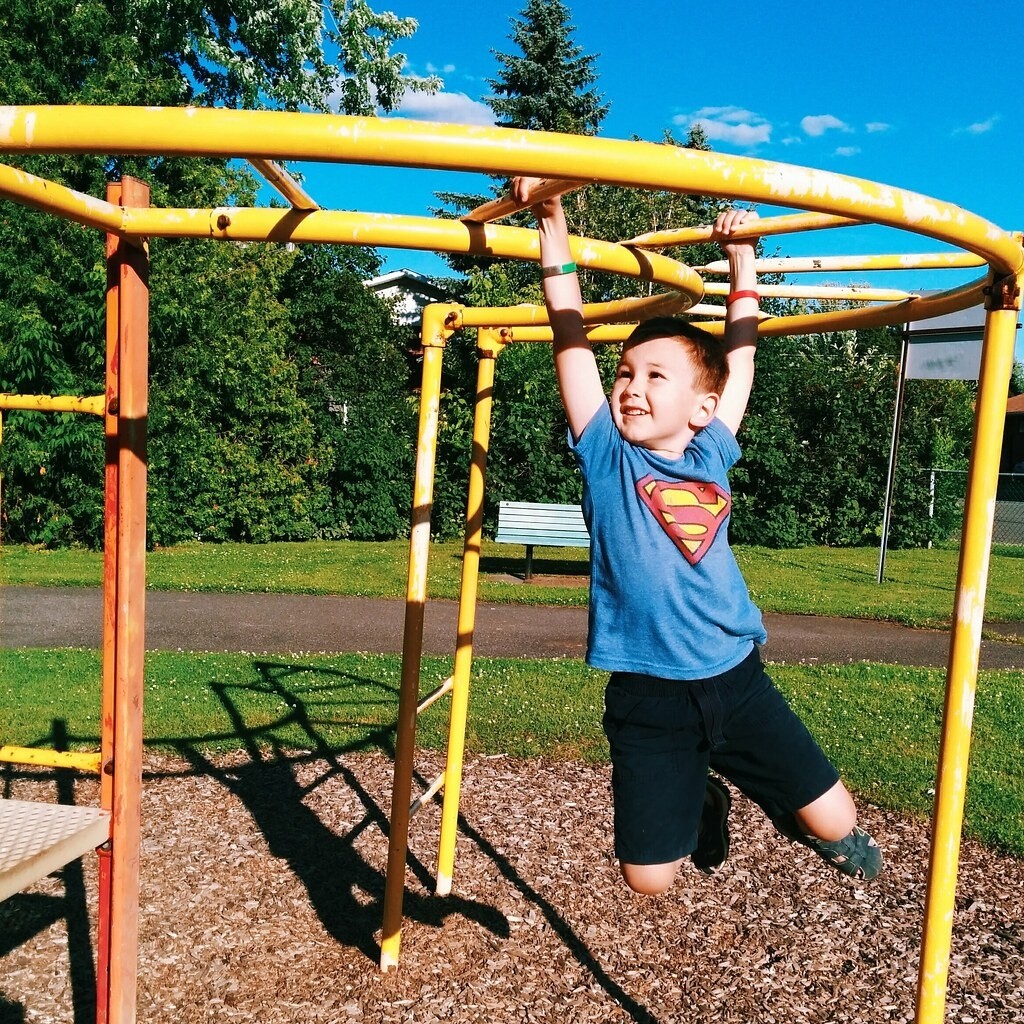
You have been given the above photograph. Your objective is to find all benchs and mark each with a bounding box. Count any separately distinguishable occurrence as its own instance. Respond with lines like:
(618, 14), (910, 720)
(494, 500), (591, 584)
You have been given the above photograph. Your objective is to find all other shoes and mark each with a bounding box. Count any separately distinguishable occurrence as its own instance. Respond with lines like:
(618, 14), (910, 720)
(691, 775), (731, 875)
(796, 824), (881, 880)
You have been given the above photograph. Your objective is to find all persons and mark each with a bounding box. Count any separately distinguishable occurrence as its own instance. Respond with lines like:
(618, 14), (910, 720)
(508, 174), (887, 897)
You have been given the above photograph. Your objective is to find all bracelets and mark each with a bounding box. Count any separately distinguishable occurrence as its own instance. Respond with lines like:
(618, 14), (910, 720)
(726, 289), (761, 307)
(540, 260), (578, 279)
